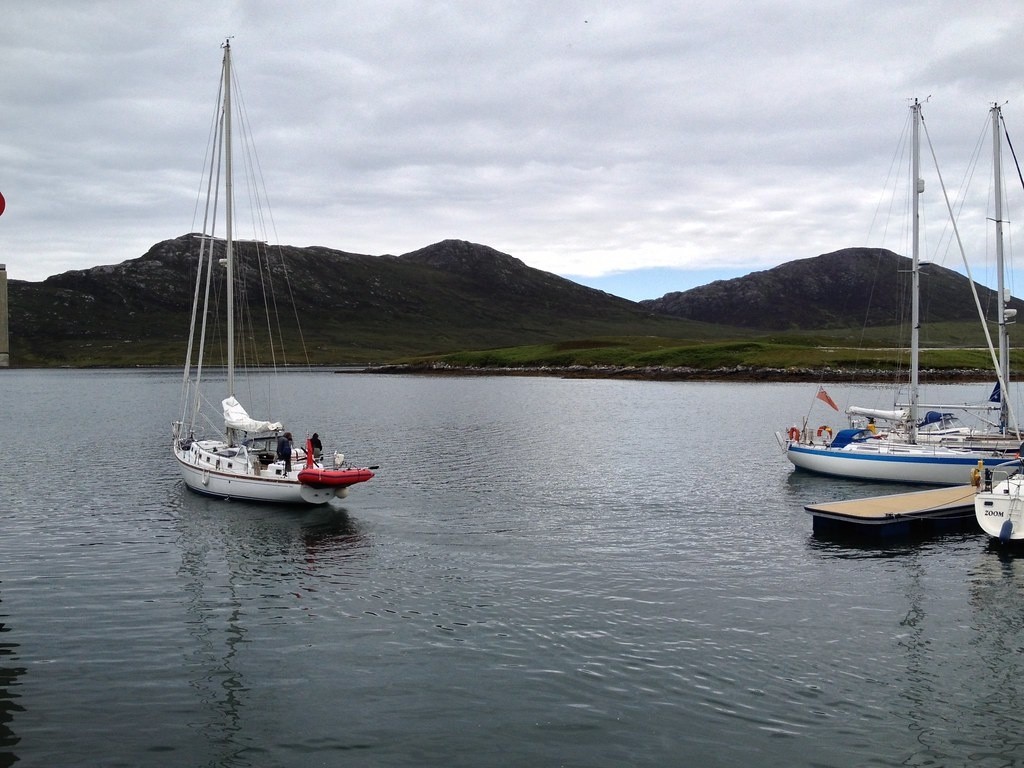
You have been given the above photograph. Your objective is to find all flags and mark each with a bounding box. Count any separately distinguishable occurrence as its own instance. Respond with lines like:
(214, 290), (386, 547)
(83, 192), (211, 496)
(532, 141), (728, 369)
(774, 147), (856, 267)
(816, 386), (839, 411)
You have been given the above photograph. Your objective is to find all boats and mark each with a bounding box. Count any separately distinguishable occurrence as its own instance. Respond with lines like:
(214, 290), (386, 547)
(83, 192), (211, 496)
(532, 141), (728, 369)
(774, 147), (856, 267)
(971, 459), (1024, 542)
(299, 465), (374, 485)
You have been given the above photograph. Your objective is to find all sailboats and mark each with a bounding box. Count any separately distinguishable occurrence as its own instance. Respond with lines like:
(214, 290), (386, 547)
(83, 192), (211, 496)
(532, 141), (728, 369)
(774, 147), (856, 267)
(847, 100), (1024, 451)
(170, 39), (378, 505)
(772, 98), (1024, 486)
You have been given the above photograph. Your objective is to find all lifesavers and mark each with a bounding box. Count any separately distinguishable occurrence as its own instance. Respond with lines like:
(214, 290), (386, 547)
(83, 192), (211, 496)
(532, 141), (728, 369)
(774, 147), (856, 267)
(817, 425), (833, 438)
(789, 427), (800, 441)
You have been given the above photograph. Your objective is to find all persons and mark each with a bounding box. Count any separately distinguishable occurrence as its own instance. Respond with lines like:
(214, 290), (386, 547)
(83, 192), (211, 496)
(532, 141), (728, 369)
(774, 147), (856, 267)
(310, 433), (323, 455)
(277, 432), (293, 471)
